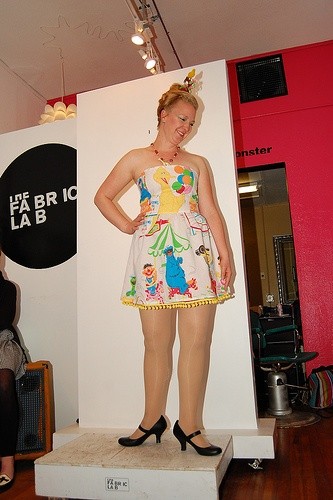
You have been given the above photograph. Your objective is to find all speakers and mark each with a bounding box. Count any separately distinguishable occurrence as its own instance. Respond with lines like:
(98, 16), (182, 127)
(14, 360), (55, 461)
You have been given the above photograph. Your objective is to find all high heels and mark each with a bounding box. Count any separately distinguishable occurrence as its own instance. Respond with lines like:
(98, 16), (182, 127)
(118, 415), (167, 447)
(173, 420), (222, 456)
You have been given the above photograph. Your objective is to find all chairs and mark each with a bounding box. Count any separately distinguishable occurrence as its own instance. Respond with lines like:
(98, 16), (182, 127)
(250, 305), (321, 429)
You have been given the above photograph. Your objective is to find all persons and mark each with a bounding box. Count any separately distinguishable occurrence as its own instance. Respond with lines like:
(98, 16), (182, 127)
(0, 271), (26, 492)
(94, 82), (237, 457)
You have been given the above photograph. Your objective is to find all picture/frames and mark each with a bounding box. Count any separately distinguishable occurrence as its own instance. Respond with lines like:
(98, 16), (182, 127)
(273, 235), (299, 305)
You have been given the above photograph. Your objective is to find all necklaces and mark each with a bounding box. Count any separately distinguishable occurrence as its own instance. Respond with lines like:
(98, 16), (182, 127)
(150, 143), (180, 166)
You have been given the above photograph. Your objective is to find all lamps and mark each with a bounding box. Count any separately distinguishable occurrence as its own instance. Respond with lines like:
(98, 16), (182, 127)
(137, 46), (157, 70)
(130, 18), (153, 45)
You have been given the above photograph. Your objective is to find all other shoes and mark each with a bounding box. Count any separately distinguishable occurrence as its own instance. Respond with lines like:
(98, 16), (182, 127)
(0, 473), (15, 489)
